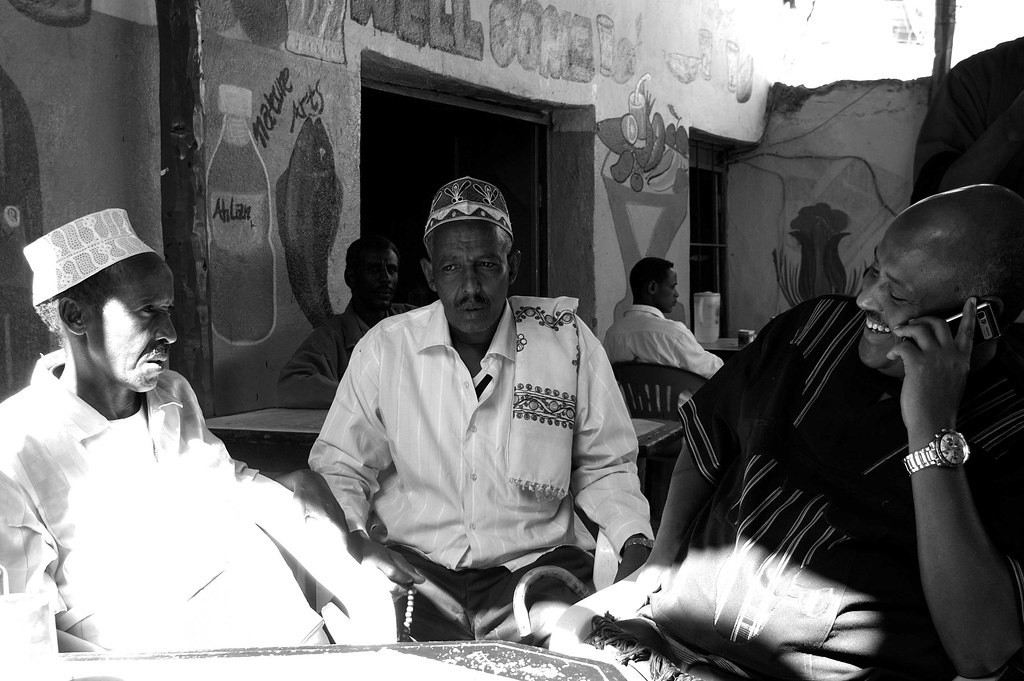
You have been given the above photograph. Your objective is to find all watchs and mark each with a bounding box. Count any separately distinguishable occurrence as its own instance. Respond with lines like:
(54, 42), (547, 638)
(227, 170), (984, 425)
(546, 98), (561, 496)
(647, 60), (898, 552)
(902, 426), (970, 477)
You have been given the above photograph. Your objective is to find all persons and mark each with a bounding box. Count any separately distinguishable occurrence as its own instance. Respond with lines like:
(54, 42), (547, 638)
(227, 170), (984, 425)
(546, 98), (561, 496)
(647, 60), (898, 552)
(274, 177), (657, 649)
(0, 207), (346, 653)
(586, 183), (1024, 681)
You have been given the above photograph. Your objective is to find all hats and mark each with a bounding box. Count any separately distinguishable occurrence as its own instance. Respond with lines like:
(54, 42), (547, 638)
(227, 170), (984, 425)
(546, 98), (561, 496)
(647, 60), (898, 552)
(422, 176), (513, 246)
(23, 209), (156, 309)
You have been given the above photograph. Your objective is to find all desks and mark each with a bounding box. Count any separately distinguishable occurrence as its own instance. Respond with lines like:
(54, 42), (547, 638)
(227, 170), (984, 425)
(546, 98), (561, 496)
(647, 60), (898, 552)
(697, 336), (741, 363)
(205, 407), (685, 497)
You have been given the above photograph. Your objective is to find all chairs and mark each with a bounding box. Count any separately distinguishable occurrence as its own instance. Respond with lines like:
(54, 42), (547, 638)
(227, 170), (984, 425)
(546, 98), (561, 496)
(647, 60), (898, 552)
(610, 361), (711, 498)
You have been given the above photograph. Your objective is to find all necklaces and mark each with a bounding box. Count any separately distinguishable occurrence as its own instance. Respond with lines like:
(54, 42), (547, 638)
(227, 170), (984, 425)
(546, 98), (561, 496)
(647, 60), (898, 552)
(398, 578), (420, 642)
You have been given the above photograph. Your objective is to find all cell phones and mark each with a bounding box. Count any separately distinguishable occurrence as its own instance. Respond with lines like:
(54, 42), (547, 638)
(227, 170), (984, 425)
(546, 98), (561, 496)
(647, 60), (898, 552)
(899, 301), (1002, 355)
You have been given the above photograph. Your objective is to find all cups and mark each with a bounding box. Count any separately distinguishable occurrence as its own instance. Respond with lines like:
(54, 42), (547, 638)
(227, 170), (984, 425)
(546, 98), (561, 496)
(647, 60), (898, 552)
(694, 292), (720, 343)
(738, 330), (755, 348)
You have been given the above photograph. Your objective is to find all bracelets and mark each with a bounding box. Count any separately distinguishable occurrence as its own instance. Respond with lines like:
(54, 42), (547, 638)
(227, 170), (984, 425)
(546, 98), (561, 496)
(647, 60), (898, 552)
(620, 537), (653, 558)
